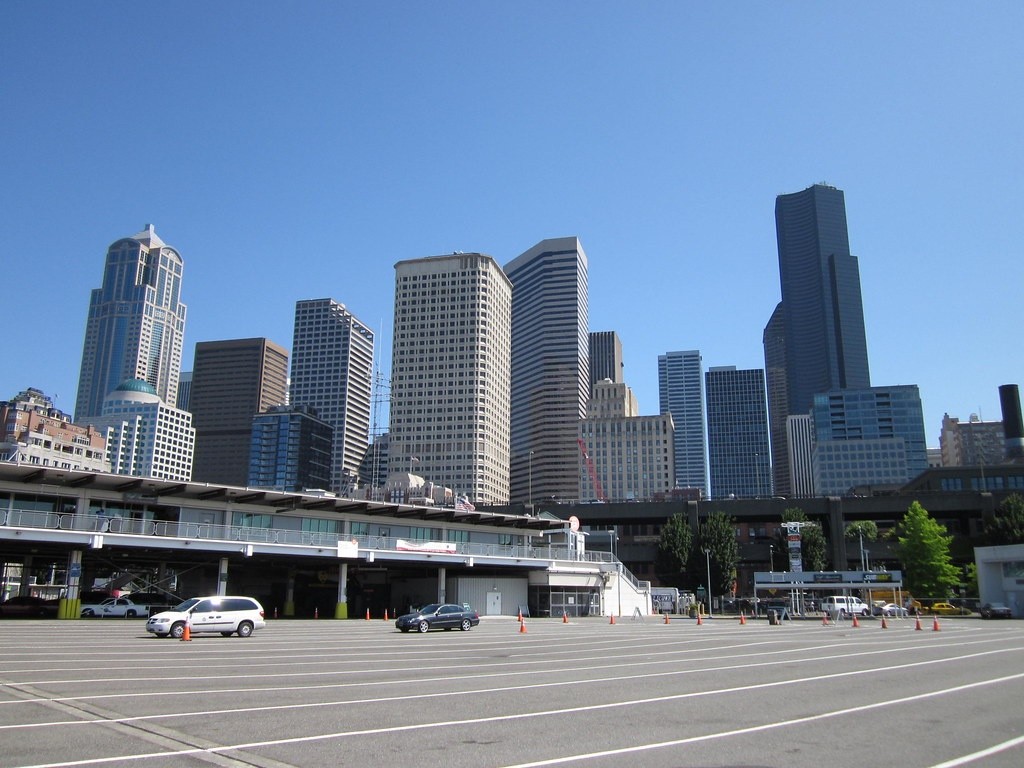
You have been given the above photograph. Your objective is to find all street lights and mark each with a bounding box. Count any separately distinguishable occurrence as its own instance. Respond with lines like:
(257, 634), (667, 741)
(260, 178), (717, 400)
(529, 450), (535, 504)
(769, 544), (775, 572)
(704, 548), (712, 618)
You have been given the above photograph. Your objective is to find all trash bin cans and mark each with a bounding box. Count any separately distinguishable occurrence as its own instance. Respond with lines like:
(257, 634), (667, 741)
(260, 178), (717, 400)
(689, 607), (697, 618)
(768, 610), (778, 625)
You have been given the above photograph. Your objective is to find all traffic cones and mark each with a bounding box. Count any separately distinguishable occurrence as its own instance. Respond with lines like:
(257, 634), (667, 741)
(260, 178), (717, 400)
(932, 616), (941, 631)
(517, 608), (526, 632)
(822, 611), (829, 625)
(852, 613), (860, 627)
(366, 608), (371, 620)
(664, 612), (670, 624)
(384, 608), (389, 621)
(179, 617), (192, 641)
(697, 612), (702, 625)
(915, 617), (923, 630)
(881, 614), (887, 628)
(314, 608), (318, 618)
(740, 611), (746, 624)
(273, 607), (277, 619)
(610, 611), (615, 624)
(563, 611), (569, 623)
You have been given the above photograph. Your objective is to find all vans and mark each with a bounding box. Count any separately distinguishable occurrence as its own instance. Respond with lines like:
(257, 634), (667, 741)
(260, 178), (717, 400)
(821, 596), (869, 616)
(145, 595), (266, 639)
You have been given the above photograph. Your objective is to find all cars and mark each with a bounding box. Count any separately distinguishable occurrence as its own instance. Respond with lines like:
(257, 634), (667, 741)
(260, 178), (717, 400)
(923, 602), (960, 616)
(0, 596), (149, 619)
(395, 603), (479, 632)
(872, 600), (909, 617)
(586, 499), (602, 503)
(980, 602), (1013, 619)
